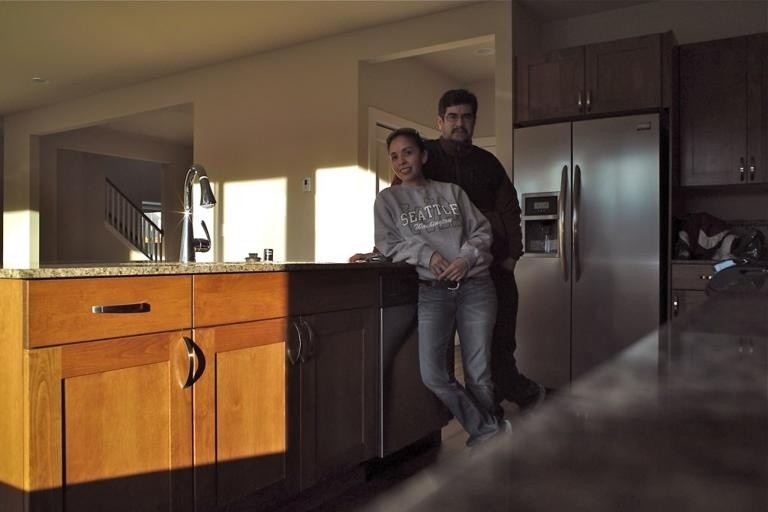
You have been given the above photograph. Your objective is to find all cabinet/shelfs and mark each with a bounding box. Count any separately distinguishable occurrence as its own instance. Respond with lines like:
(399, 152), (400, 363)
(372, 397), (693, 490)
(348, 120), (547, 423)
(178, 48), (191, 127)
(1, 279), (192, 510)
(195, 267), (381, 509)
(671, 260), (719, 321)
(515, 31), (664, 127)
(674, 30), (767, 188)
(668, 331), (767, 487)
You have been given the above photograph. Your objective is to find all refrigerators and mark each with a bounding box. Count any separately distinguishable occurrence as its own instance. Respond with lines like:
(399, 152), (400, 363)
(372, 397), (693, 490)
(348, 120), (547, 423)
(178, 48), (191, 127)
(511, 106), (667, 425)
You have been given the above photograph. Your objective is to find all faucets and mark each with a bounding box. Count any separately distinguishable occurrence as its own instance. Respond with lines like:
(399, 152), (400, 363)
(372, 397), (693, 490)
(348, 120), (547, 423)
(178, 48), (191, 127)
(179, 165), (215, 262)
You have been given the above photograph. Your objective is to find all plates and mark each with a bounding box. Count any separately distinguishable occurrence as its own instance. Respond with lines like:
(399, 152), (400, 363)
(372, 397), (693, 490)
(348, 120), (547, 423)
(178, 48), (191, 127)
(245, 258), (260, 262)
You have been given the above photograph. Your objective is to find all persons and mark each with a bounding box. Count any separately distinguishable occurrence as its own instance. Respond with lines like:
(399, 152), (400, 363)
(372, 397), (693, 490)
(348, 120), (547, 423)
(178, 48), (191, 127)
(373, 128), (512, 458)
(350, 89), (545, 413)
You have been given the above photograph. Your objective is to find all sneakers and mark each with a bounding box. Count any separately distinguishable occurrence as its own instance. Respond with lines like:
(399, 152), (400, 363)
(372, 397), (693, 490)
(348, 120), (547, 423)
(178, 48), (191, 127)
(519, 383), (546, 418)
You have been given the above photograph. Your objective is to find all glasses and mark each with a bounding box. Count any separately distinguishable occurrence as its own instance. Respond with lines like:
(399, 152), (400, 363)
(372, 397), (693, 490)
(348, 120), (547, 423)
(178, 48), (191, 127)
(444, 114), (473, 122)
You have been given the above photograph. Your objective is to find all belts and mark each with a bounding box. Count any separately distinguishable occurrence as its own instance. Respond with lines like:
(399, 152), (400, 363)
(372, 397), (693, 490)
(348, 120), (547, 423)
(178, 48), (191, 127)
(418, 278), (463, 291)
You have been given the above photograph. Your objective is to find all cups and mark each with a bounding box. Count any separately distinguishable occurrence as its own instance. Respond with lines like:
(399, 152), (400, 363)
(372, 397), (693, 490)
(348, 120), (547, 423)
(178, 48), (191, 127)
(249, 253), (257, 258)
(264, 248), (273, 262)
